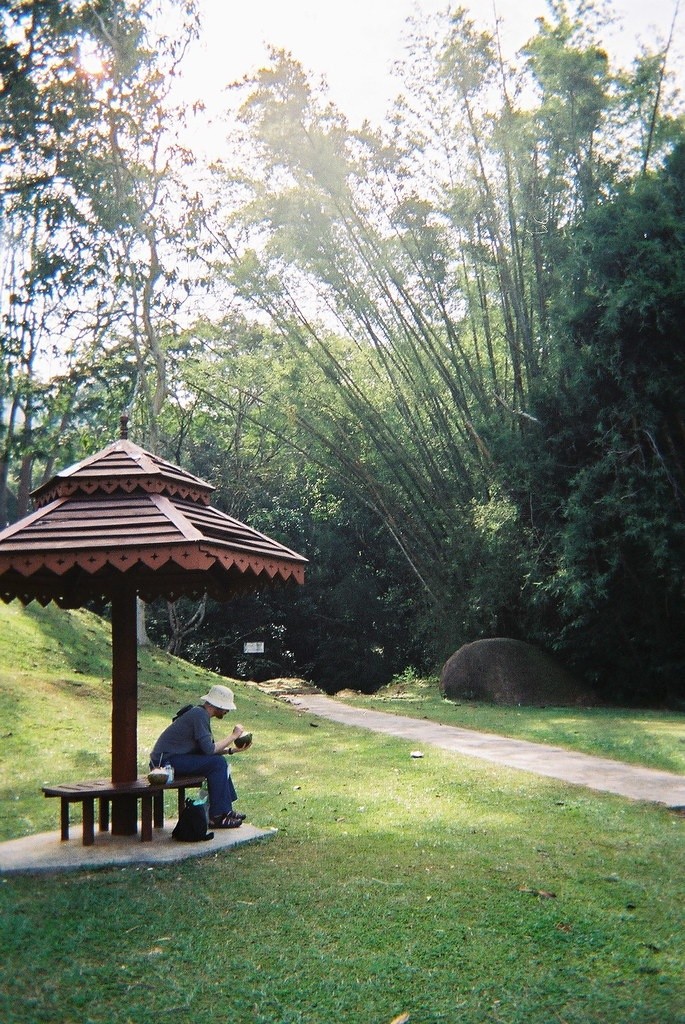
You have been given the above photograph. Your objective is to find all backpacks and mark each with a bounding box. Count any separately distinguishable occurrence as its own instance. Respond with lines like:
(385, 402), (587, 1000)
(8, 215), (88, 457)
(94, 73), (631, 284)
(172, 797), (214, 842)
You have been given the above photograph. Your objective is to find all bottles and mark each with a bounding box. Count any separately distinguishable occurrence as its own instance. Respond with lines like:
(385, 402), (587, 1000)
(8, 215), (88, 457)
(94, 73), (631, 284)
(164, 765), (174, 784)
(199, 780), (208, 804)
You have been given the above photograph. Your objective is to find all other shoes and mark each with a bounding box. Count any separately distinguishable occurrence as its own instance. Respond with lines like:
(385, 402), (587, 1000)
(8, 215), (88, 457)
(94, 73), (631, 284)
(208, 815), (242, 828)
(226, 810), (246, 820)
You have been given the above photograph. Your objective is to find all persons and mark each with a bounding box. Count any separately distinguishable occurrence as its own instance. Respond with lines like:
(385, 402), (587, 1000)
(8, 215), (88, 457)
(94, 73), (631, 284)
(148, 685), (253, 829)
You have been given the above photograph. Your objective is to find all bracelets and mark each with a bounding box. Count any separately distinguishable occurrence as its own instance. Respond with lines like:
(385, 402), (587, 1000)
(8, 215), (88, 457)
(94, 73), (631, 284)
(228, 747), (233, 755)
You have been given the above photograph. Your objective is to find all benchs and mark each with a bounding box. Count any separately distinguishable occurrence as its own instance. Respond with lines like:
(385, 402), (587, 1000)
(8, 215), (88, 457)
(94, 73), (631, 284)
(42, 775), (202, 843)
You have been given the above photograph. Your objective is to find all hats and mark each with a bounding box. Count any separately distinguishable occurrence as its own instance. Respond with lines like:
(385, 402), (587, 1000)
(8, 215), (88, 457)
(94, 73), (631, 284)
(201, 685), (236, 709)
